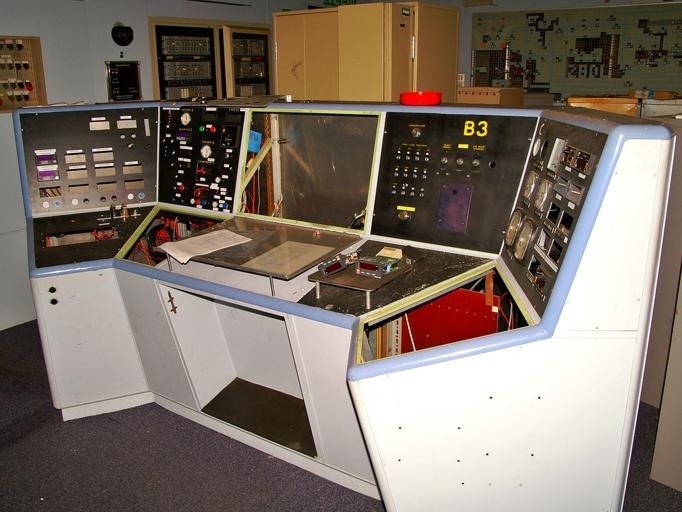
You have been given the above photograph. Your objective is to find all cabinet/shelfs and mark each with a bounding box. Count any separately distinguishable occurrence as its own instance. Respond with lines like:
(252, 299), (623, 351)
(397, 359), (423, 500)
(273, 4), (461, 107)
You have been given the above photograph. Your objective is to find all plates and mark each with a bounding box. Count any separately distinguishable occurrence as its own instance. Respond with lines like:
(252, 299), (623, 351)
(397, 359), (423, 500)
(400, 92), (442, 106)
